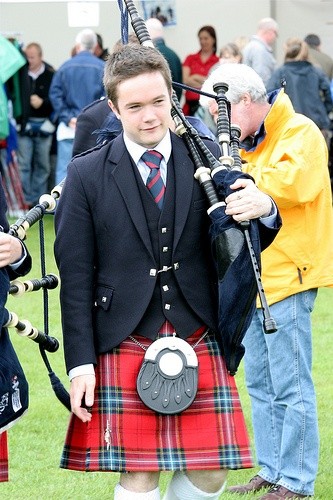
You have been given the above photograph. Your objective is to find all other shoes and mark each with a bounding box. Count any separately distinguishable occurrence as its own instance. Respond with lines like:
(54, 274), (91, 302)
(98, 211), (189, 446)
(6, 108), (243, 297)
(261, 485), (311, 500)
(227, 474), (272, 495)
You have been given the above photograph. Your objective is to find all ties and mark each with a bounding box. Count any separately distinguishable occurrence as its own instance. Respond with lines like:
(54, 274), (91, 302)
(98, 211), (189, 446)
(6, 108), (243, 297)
(141, 151), (166, 210)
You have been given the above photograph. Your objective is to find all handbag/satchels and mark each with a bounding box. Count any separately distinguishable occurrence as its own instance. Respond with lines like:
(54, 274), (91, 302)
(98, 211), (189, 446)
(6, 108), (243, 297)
(136, 331), (199, 415)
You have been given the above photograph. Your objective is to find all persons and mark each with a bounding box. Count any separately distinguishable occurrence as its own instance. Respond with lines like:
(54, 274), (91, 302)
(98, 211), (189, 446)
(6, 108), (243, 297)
(304, 34), (333, 82)
(53, 45), (283, 500)
(73, 36), (141, 157)
(45, 29), (109, 213)
(0, 184), (32, 483)
(266, 38), (333, 144)
(182, 26), (219, 116)
(143, 19), (181, 101)
(15, 43), (54, 208)
(199, 63), (333, 500)
(240, 17), (280, 88)
